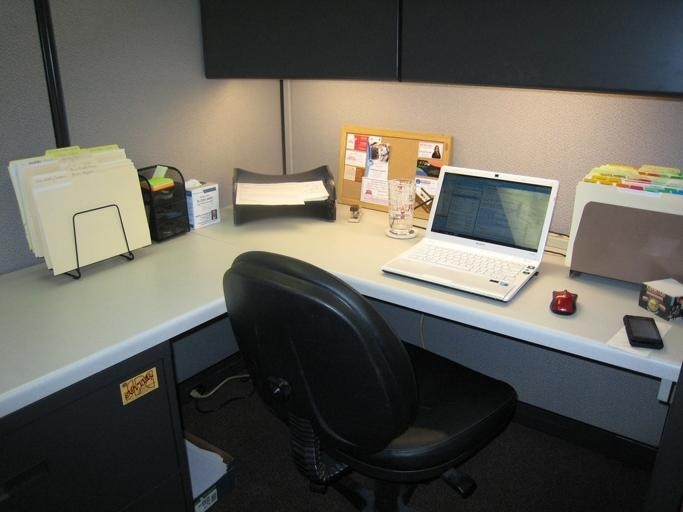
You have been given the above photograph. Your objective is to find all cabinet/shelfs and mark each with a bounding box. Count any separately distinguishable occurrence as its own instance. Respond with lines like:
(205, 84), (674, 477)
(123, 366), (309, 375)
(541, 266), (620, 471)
(193, 0), (682, 101)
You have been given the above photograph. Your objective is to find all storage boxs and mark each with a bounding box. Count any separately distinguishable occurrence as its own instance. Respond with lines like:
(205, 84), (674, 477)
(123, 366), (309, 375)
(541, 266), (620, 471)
(181, 426), (239, 511)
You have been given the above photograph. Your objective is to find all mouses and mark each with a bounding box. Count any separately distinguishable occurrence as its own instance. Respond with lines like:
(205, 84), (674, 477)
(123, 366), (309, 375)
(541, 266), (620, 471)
(550, 290), (577, 315)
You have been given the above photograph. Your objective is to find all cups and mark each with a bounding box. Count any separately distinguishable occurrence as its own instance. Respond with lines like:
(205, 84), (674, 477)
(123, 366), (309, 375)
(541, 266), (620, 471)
(386, 178), (418, 234)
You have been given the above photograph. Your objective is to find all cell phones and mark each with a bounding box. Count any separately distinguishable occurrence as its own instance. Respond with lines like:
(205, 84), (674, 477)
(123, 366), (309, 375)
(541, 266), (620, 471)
(623, 315), (664, 350)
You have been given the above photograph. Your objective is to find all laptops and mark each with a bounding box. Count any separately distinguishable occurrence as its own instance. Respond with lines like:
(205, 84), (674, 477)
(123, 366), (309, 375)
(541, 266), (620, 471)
(381, 165), (560, 304)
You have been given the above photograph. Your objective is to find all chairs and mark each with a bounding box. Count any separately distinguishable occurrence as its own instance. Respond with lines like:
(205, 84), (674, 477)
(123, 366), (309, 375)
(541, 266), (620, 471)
(217, 249), (521, 511)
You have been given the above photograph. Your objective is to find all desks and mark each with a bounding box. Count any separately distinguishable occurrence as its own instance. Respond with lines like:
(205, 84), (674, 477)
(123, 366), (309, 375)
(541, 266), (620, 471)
(0, 205), (683, 511)
